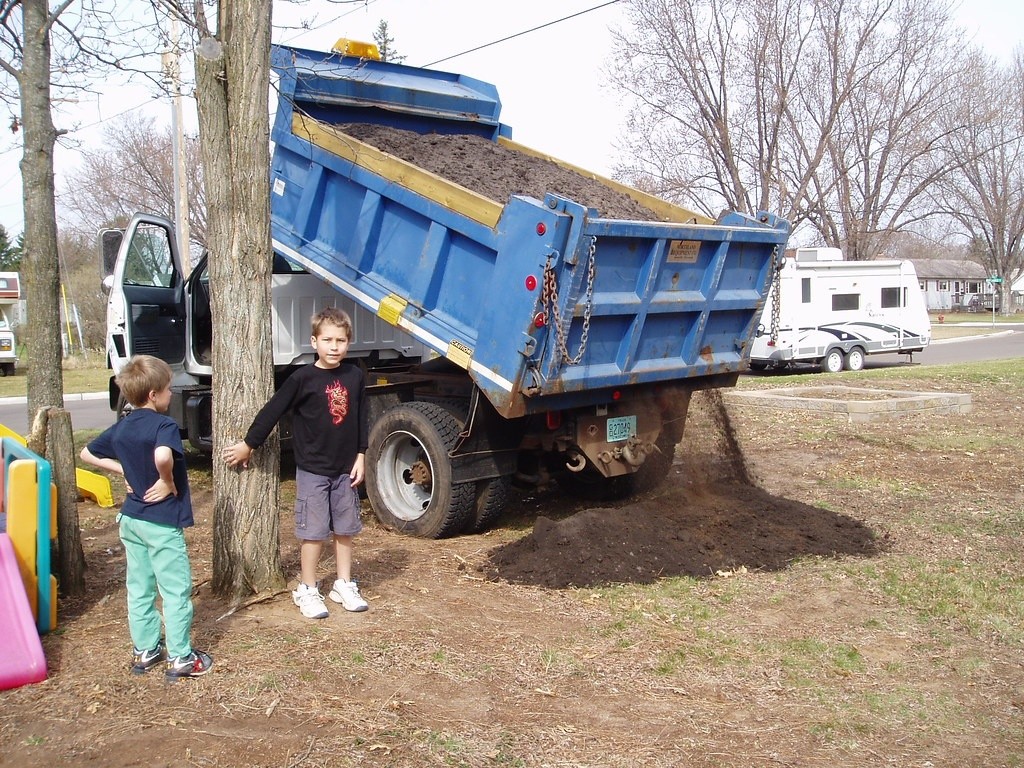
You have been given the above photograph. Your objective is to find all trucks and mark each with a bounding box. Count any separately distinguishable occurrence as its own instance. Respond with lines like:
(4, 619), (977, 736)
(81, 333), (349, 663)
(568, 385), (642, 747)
(95, 39), (795, 544)
(0, 272), (22, 377)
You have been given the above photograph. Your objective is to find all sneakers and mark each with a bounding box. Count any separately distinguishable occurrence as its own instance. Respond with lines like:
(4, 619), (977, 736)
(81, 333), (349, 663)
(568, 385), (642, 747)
(131, 643), (166, 675)
(292, 581), (329, 620)
(167, 648), (212, 678)
(329, 579), (369, 612)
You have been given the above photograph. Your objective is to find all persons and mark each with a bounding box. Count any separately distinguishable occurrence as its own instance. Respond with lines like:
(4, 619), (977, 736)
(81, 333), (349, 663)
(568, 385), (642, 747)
(221, 308), (369, 618)
(80, 354), (214, 682)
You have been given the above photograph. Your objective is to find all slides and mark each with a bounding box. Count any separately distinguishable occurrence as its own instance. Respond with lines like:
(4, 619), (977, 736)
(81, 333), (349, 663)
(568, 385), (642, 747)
(1, 530), (52, 692)
(0, 422), (114, 507)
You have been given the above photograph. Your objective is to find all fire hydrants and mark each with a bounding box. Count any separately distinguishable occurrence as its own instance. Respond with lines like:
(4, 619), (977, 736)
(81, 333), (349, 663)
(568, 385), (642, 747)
(938, 314), (944, 323)
